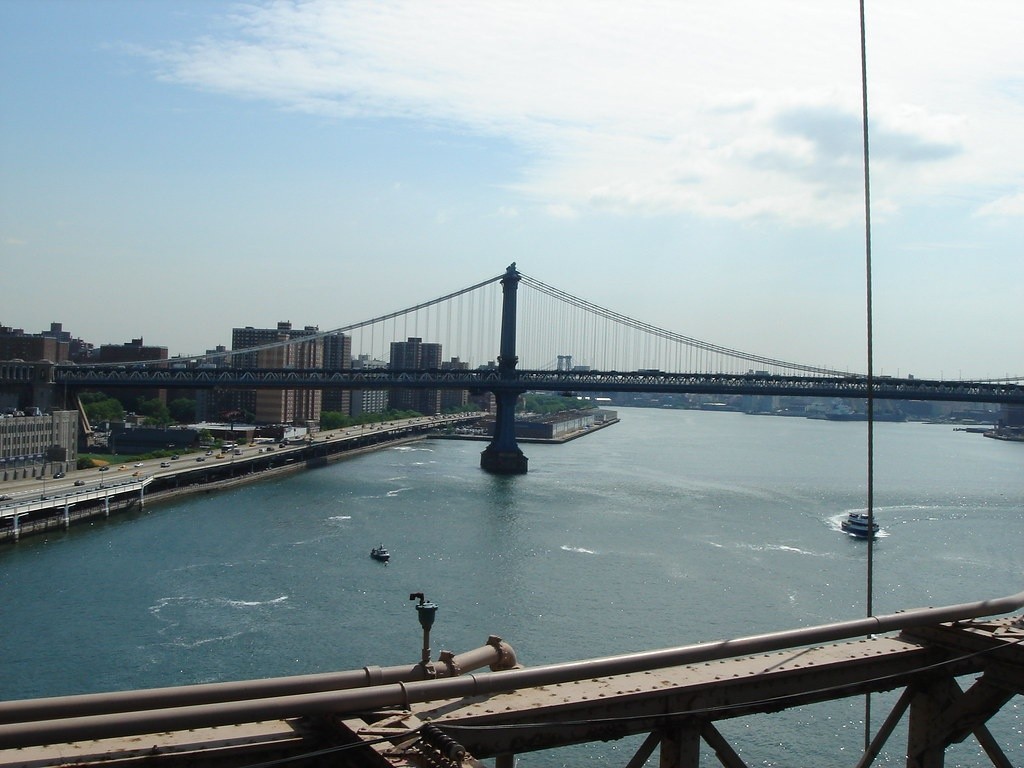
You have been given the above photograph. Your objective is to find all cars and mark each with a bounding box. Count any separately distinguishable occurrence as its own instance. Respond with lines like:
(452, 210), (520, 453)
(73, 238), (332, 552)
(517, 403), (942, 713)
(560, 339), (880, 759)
(53, 472), (65, 479)
(161, 411), (482, 468)
(74, 480), (85, 486)
(134, 463), (143, 467)
(99, 466), (109, 471)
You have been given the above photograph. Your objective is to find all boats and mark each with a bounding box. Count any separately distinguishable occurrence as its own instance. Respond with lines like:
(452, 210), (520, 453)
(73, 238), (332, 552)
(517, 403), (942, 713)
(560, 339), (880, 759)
(370, 542), (391, 563)
(841, 511), (879, 537)
